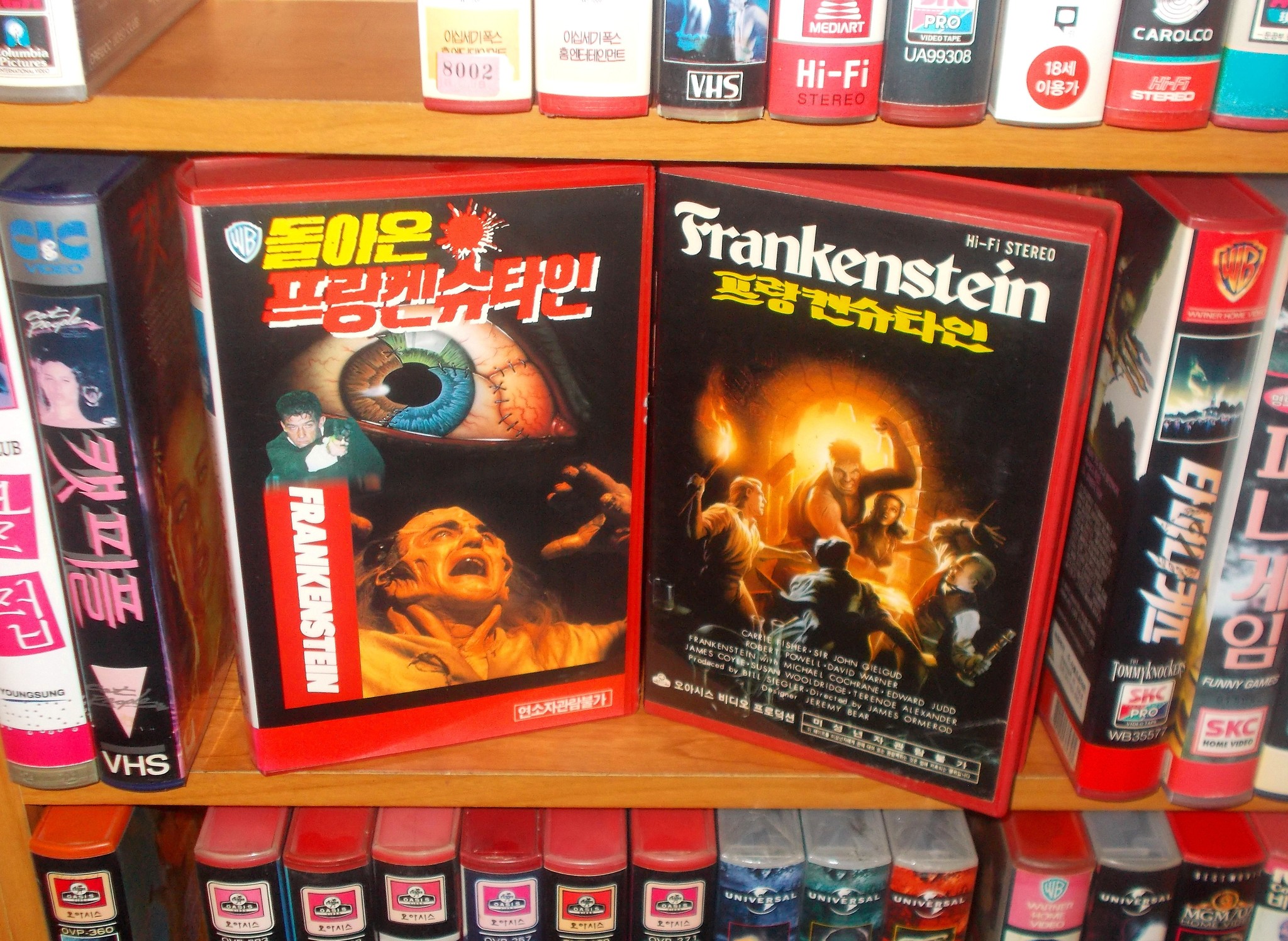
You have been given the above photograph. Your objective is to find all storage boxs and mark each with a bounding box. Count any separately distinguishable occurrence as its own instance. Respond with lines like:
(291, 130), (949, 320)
(1, 2), (1288, 939)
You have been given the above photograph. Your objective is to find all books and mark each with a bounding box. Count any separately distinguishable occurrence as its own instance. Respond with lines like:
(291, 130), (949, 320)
(2, 148), (1286, 941)
(0, 0), (205, 103)
(417, 0), (1287, 135)
